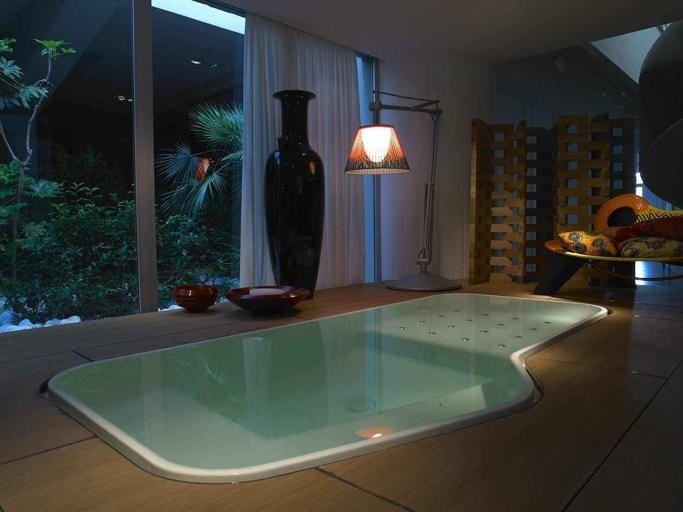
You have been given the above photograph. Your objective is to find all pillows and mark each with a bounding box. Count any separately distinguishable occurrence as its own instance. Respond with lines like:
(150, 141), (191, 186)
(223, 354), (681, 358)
(558, 214), (683, 258)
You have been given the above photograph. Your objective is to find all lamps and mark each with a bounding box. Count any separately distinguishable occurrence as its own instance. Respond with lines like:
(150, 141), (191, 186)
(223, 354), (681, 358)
(344, 89), (462, 291)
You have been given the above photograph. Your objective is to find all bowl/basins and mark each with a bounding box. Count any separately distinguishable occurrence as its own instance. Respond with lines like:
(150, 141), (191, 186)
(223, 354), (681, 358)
(172, 285), (219, 313)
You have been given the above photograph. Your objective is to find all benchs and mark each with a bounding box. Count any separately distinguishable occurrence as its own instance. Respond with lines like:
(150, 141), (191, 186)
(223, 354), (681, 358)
(544, 194), (683, 294)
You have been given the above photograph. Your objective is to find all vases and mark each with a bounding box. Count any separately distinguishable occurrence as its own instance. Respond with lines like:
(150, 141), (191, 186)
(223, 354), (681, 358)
(265, 90), (325, 299)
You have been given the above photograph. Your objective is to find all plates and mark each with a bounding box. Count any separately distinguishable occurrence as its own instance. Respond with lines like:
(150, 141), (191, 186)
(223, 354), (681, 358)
(223, 283), (311, 315)
